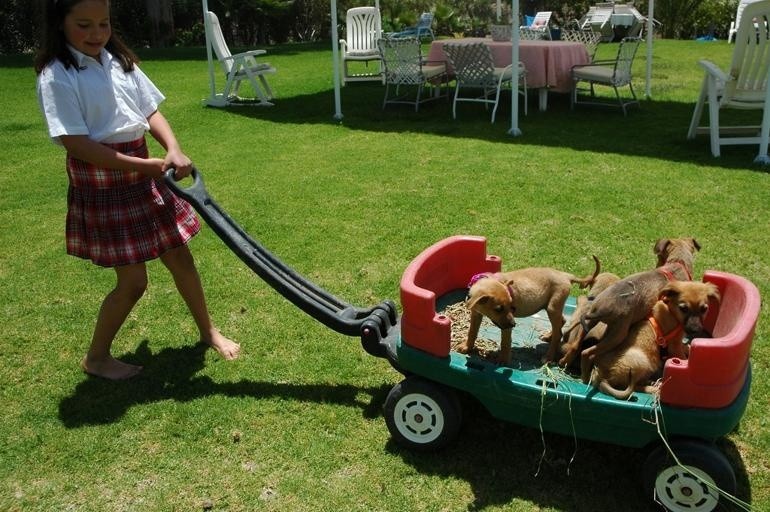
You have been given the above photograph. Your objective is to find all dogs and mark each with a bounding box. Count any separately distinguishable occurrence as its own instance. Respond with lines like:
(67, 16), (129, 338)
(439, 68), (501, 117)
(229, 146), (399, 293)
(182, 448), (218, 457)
(557, 238), (701, 384)
(457, 255), (601, 366)
(590, 280), (721, 400)
(560, 273), (620, 367)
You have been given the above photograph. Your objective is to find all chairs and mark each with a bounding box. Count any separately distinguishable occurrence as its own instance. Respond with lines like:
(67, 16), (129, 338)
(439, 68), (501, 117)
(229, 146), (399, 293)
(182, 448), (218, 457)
(338, 7), (386, 87)
(560, 30), (602, 64)
(388, 13), (436, 47)
(376, 38), (450, 114)
(728, 9), (768, 45)
(687, 0), (770, 157)
(442, 41), (528, 123)
(520, 26), (552, 41)
(489, 25), (516, 41)
(202, 12), (276, 107)
(570, 37), (642, 116)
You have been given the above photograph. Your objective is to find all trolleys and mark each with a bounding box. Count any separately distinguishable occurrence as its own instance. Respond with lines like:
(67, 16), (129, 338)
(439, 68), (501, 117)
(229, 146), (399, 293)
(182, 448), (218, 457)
(161, 161), (763, 511)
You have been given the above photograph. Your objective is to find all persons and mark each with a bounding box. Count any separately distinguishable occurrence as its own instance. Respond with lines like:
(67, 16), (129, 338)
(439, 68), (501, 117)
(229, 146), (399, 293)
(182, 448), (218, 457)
(34, 0), (239, 380)
